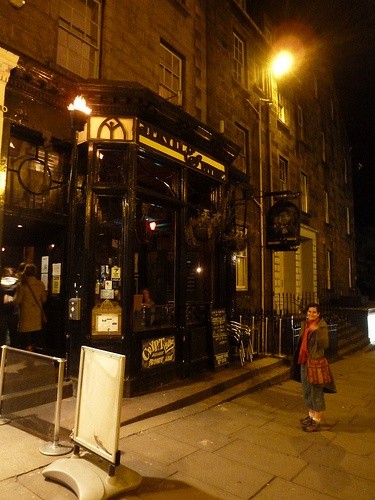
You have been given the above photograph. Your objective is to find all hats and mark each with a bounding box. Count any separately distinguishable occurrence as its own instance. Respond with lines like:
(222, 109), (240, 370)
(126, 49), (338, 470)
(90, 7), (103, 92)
(24, 264), (36, 274)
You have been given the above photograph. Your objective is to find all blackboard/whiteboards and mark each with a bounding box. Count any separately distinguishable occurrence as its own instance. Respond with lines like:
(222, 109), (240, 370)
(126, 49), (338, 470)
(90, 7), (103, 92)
(69, 344), (125, 469)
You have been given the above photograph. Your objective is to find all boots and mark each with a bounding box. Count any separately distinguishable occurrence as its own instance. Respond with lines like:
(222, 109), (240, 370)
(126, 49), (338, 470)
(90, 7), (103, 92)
(24, 345), (35, 368)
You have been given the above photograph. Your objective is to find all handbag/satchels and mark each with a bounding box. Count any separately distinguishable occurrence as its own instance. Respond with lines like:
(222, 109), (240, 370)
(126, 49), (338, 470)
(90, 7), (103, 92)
(307, 357), (330, 384)
(42, 311), (47, 328)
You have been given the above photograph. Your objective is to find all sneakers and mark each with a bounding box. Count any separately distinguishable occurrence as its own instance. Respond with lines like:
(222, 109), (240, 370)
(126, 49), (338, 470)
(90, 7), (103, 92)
(300, 416), (320, 432)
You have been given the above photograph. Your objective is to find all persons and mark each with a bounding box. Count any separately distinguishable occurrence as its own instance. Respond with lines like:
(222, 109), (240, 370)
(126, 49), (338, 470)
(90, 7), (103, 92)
(0, 263), (25, 347)
(10, 264), (47, 352)
(291, 303), (337, 432)
(139, 289), (155, 337)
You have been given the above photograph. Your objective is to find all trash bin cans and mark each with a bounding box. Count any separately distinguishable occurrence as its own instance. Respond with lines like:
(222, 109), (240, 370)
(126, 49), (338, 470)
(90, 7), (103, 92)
(291, 312), (339, 359)
